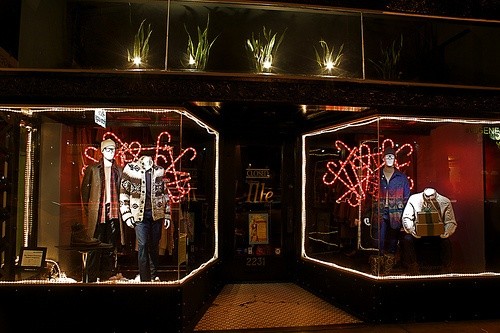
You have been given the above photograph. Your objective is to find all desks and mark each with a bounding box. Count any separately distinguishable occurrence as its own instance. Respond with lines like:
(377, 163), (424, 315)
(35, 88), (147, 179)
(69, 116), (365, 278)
(55, 243), (112, 284)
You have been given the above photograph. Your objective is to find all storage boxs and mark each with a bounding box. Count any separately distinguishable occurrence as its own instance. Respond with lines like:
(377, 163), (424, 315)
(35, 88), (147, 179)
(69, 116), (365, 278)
(416, 211), (444, 236)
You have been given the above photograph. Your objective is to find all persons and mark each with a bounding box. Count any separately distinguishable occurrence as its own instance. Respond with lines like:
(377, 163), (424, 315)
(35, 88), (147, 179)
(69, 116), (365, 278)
(80, 137), (125, 283)
(119, 153), (174, 281)
(368, 146), (458, 274)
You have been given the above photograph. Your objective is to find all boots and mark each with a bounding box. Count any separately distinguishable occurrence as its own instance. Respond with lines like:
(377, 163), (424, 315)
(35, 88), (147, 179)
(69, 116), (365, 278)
(71, 223), (101, 247)
(381, 253), (396, 276)
(370, 255), (383, 276)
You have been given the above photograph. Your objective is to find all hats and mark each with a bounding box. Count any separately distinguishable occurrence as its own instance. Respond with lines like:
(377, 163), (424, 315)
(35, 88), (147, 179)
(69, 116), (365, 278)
(101, 139), (116, 152)
(383, 148), (394, 158)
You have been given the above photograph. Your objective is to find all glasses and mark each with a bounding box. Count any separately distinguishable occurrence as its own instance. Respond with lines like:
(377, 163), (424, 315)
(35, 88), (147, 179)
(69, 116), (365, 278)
(104, 147), (115, 152)
(384, 155), (394, 159)
